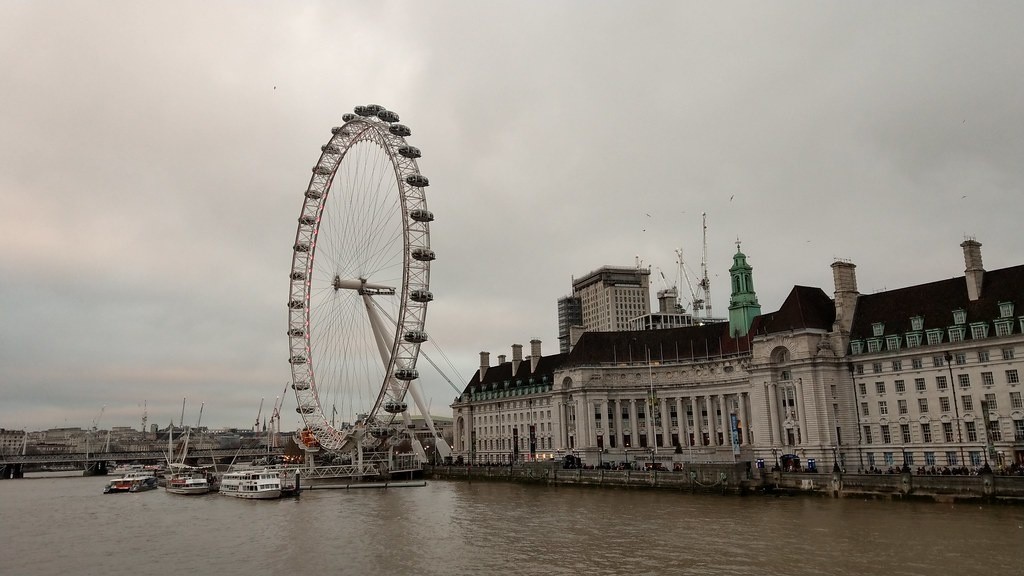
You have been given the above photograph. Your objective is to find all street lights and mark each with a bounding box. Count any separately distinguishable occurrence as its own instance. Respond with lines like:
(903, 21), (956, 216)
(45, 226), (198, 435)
(624, 450), (628, 468)
(599, 447), (603, 468)
(652, 448), (655, 469)
(832, 447), (840, 472)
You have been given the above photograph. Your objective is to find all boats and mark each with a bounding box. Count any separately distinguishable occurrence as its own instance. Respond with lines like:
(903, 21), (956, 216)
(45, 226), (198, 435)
(124, 470), (162, 478)
(106, 465), (161, 476)
(164, 427), (210, 494)
(217, 442), (282, 499)
(103, 478), (158, 495)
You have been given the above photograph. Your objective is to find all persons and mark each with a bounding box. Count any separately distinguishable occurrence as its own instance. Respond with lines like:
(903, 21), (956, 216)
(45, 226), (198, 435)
(782, 465), (802, 474)
(856, 462), (1024, 477)
(436, 459), (512, 467)
(563, 458), (683, 472)
